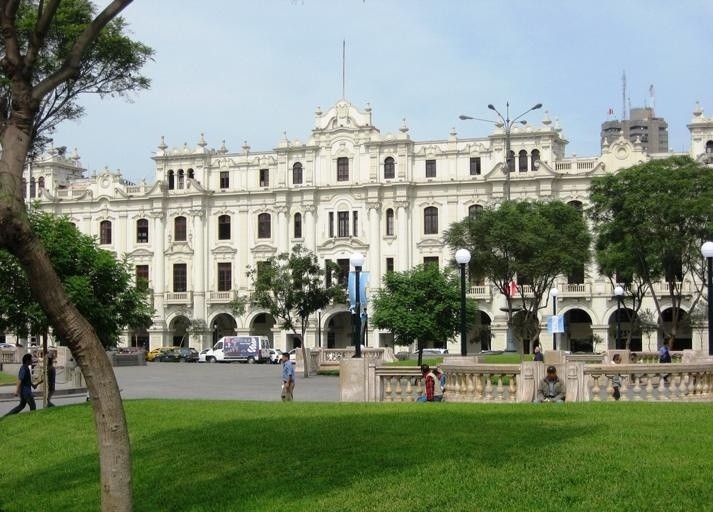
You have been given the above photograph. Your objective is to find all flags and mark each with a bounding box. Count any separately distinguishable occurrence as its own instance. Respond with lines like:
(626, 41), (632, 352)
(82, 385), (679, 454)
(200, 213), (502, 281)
(500, 280), (516, 295)
(547, 314), (565, 333)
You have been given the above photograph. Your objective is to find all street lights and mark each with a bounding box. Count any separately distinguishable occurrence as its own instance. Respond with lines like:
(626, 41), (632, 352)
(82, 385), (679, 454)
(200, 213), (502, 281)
(348, 251), (366, 358)
(612, 286), (625, 349)
(700, 240), (712, 356)
(456, 101), (544, 205)
(454, 248), (472, 355)
(549, 288), (560, 349)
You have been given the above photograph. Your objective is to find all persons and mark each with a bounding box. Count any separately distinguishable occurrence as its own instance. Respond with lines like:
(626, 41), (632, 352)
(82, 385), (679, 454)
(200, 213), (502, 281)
(659, 336), (672, 363)
(629, 353), (645, 383)
(433, 369), (446, 394)
(33, 350), (58, 407)
(608, 353), (623, 400)
(534, 346), (543, 361)
(225, 338), (249, 353)
(419, 364), (444, 402)
(279, 352), (296, 400)
(533, 365), (567, 403)
(0, 352), (37, 420)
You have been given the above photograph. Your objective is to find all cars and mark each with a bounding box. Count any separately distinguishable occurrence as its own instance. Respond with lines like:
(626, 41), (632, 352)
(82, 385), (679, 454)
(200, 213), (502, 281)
(118, 335), (297, 363)
(412, 349), (515, 358)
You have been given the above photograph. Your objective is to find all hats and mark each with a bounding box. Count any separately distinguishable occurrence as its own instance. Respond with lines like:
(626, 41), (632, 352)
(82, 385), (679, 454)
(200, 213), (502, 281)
(547, 366), (556, 373)
